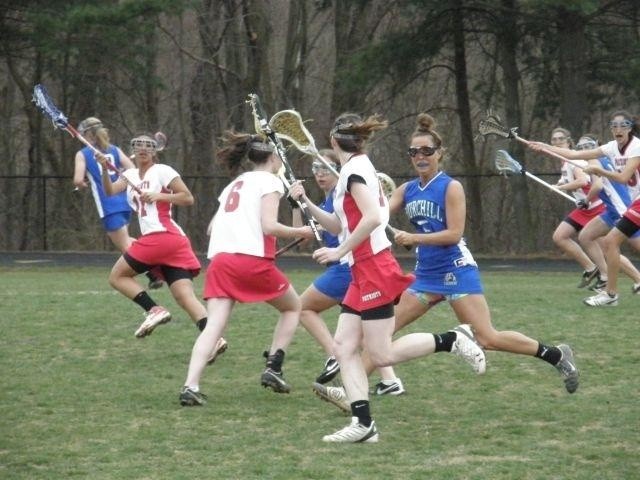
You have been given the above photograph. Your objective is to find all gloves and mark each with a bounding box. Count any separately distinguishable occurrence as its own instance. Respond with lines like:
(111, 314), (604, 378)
(575, 197), (589, 210)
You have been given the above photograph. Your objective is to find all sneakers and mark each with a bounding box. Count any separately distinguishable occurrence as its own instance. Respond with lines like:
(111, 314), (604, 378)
(368, 382), (405, 397)
(448, 323), (486, 377)
(554, 343), (580, 394)
(147, 273), (164, 289)
(179, 386), (208, 406)
(323, 415), (378, 445)
(207, 336), (228, 366)
(578, 267), (640, 308)
(316, 357), (340, 384)
(260, 368), (290, 393)
(310, 380), (353, 414)
(134, 306), (172, 338)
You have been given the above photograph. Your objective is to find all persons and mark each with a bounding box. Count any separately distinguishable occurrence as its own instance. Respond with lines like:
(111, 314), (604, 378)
(73, 116), (163, 289)
(93, 132), (227, 365)
